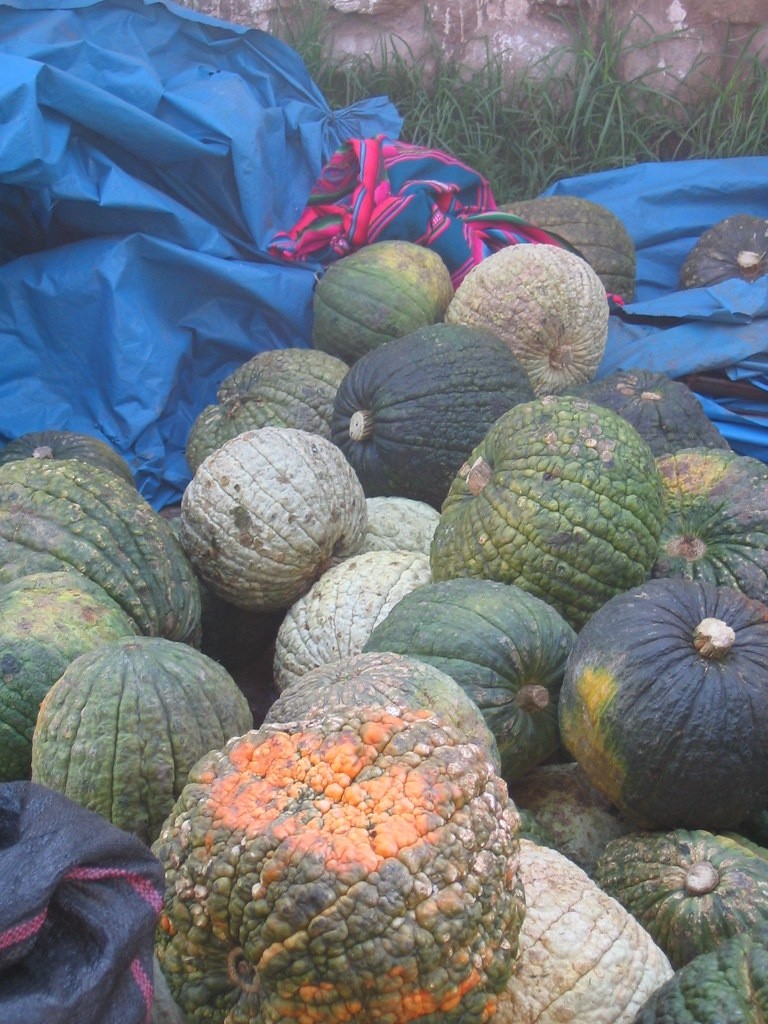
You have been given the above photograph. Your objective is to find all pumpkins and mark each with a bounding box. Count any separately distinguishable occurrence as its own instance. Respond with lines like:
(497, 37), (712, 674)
(0, 200), (768, 1024)
